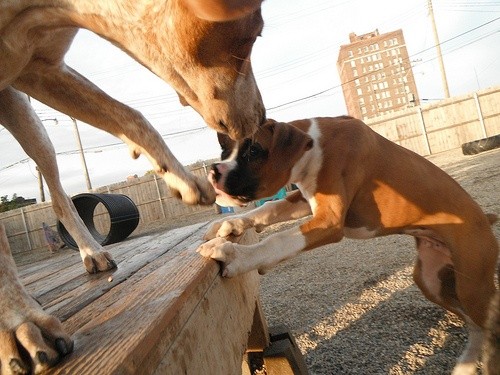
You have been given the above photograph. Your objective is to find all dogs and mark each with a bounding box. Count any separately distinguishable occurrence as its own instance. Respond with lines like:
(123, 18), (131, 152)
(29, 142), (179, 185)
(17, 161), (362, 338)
(197, 116), (500, 375)
(0, 0), (266, 375)
